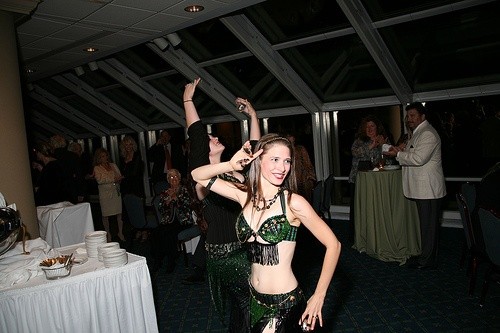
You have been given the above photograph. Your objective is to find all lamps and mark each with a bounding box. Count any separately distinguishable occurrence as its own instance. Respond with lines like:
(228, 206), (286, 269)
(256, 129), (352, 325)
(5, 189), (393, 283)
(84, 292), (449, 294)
(151, 33), (183, 52)
(74, 61), (100, 76)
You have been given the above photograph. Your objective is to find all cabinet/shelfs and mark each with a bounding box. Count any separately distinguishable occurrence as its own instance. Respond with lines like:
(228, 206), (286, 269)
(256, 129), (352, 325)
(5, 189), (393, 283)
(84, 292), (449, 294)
(34, 201), (95, 244)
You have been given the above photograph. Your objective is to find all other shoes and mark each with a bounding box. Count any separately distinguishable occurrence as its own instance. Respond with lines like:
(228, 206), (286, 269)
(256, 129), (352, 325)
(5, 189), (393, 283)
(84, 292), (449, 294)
(107, 233), (113, 241)
(182, 271), (204, 283)
(167, 262), (177, 274)
(406, 257), (421, 266)
(136, 231), (141, 239)
(117, 232), (127, 242)
(349, 224), (354, 232)
(142, 231), (148, 240)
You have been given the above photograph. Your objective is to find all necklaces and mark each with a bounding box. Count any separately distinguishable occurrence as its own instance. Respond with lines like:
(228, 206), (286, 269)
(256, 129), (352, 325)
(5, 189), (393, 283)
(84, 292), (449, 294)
(250, 185), (288, 210)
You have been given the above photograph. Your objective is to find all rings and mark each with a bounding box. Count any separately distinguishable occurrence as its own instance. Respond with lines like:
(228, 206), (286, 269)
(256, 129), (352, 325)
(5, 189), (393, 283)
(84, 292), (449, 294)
(313, 316), (319, 320)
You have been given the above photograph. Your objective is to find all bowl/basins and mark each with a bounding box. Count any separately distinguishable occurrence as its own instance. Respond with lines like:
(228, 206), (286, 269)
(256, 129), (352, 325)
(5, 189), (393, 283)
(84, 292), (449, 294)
(42, 264), (72, 280)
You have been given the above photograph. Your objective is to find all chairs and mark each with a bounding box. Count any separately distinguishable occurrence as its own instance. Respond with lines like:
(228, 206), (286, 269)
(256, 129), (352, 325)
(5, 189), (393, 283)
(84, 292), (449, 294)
(456, 192), (481, 298)
(472, 206), (500, 305)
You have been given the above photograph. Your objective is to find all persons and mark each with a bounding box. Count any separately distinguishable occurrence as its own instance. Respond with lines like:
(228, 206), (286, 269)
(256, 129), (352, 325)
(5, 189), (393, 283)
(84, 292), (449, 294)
(382, 102), (446, 269)
(34, 128), (206, 280)
(348, 115), (392, 222)
(394, 114), (415, 151)
(191, 133), (337, 333)
(178, 76), (266, 331)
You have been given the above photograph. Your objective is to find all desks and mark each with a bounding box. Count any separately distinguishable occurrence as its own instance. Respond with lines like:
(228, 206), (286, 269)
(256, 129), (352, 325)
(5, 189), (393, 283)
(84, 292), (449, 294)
(0, 239), (161, 333)
(355, 170), (422, 264)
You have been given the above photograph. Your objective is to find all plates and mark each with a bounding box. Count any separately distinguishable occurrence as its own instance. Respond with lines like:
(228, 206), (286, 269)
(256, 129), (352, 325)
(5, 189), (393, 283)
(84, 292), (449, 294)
(85, 230), (127, 269)
(383, 164), (399, 170)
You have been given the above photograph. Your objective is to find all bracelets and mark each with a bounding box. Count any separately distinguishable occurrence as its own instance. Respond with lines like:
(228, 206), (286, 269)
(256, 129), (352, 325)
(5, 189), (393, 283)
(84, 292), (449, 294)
(205, 174), (216, 190)
(183, 98), (192, 104)
